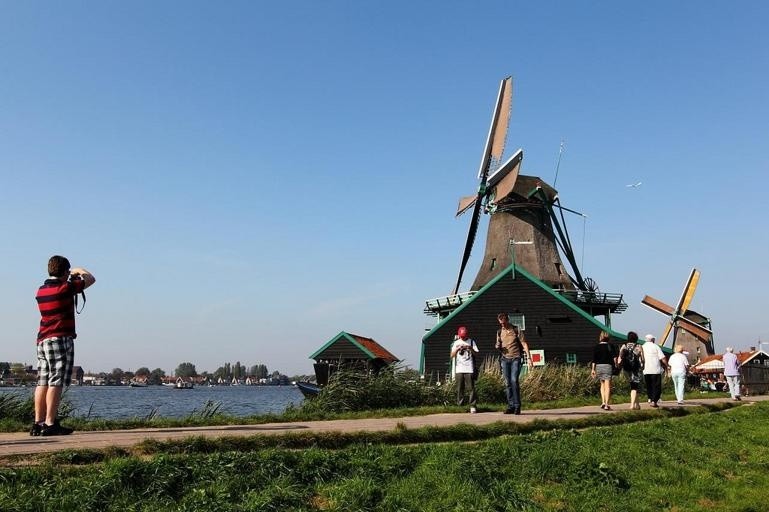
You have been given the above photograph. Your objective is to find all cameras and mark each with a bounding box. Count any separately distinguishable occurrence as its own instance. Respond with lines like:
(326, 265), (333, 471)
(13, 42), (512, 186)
(69, 275), (81, 282)
(459, 349), (464, 355)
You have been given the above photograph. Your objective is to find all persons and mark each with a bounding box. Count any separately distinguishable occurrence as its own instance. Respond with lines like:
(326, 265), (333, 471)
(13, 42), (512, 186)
(589, 330), (620, 410)
(448, 327), (480, 414)
(616, 331), (647, 413)
(27, 256), (95, 438)
(667, 344), (690, 405)
(494, 310), (533, 416)
(639, 333), (671, 408)
(722, 345), (744, 402)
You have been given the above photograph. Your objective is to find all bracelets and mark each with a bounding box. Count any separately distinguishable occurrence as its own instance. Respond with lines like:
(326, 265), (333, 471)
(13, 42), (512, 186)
(527, 357), (531, 360)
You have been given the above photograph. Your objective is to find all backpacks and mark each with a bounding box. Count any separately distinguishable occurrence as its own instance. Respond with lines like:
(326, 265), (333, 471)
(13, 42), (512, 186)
(621, 344), (639, 372)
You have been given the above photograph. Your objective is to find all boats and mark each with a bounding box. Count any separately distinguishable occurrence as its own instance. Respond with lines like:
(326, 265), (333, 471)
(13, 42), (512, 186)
(130, 377), (298, 389)
(297, 330), (401, 405)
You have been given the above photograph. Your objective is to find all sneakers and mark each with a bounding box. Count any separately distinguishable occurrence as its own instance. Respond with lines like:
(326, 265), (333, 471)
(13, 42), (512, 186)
(503, 409), (511, 414)
(514, 409), (520, 414)
(471, 408), (476, 414)
(30, 423), (41, 436)
(41, 423), (73, 436)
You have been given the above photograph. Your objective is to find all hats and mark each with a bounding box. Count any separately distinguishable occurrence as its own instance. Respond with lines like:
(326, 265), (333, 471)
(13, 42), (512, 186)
(458, 326), (468, 337)
